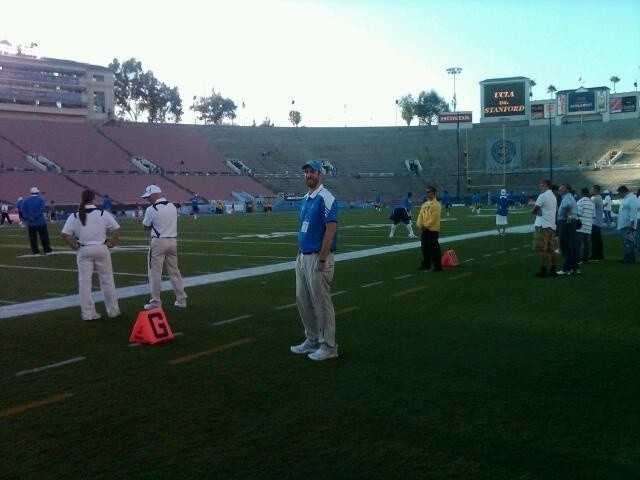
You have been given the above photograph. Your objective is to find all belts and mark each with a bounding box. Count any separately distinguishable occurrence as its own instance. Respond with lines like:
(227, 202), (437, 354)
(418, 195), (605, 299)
(300, 250), (321, 255)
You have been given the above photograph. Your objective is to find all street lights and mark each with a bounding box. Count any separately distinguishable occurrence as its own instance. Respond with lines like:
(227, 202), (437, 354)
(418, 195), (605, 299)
(446, 68), (462, 111)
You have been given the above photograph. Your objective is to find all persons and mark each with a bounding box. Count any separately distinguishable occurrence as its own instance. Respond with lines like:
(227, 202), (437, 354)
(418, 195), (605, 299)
(263, 202), (268, 213)
(375, 192), (381, 212)
(140, 184), (188, 310)
(531, 178), (640, 278)
(442, 189), (453, 216)
(0, 186), (56, 255)
(192, 197), (199, 219)
(289, 159), (340, 363)
(120, 201), (147, 218)
(268, 202), (272, 212)
(248, 204), (253, 212)
(60, 188), (122, 322)
(102, 194), (120, 213)
(403, 192), (413, 223)
(175, 202), (181, 216)
(416, 185), (442, 273)
(389, 204), (417, 239)
(214, 200), (223, 216)
(468, 188), (525, 236)
(232, 203), (234, 212)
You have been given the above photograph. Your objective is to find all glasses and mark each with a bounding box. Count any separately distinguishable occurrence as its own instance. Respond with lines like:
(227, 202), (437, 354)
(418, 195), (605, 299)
(425, 191), (433, 194)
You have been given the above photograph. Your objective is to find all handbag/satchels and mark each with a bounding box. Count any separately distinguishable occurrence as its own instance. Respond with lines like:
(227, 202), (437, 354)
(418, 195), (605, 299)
(576, 219), (582, 230)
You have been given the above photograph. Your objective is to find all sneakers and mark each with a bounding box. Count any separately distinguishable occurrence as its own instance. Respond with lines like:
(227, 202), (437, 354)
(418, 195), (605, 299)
(588, 257), (600, 261)
(175, 300), (187, 308)
(418, 266), (431, 270)
(308, 343), (338, 361)
(548, 270), (557, 277)
(408, 235), (418, 239)
(291, 339), (320, 354)
(533, 271), (547, 278)
(89, 313), (101, 320)
(557, 271), (571, 277)
(144, 303), (161, 310)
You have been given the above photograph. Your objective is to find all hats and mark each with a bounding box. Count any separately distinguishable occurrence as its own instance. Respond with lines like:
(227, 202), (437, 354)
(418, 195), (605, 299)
(603, 190), (610, 194)
(140, 185), (162, 198)
(501, 189), (506, 196)
(301, 160), (320, 171)
(30, 187), (40, 193)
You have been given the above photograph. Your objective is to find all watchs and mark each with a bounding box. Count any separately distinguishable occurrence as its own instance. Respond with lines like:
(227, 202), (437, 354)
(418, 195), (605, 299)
(319, 258), (327, 263)
